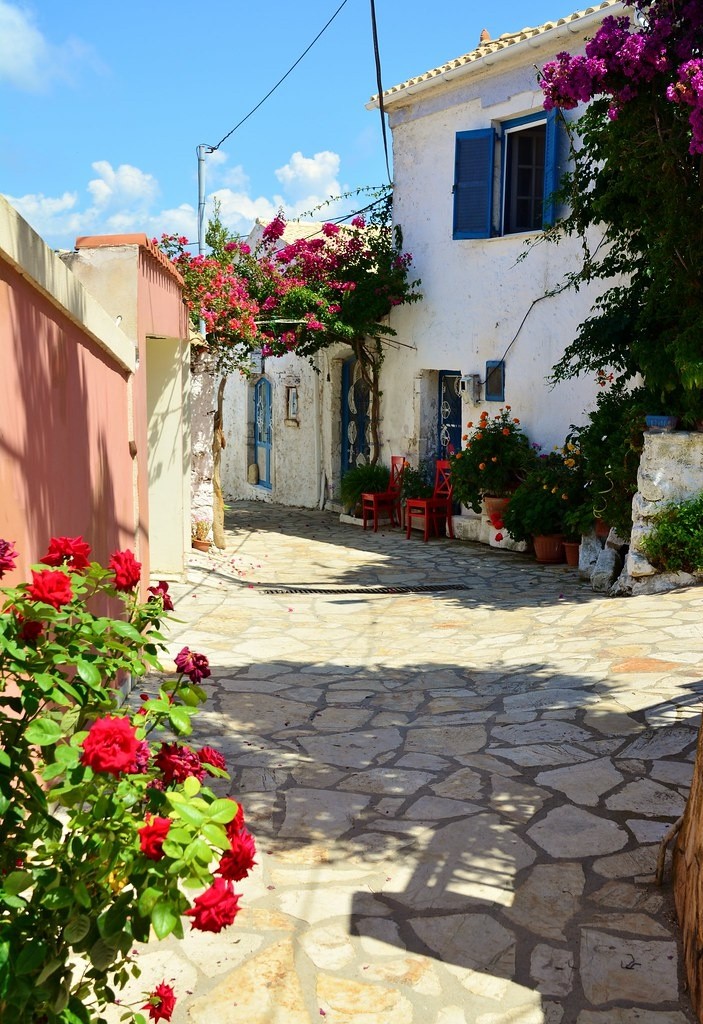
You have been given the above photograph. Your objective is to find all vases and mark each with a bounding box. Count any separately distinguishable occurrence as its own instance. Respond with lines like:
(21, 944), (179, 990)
(560, 541), (579, 566)
(340, 513), (396, 527)
(594, 517), (610, 537)
(645, 415), (677, 433)
(531, 533), (564, 560)
(484, 495), (510, 520)
(404, 508), (425, 530)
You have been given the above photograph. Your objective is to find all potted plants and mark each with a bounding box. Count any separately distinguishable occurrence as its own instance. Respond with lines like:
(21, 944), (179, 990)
(191, 521), (212, 551)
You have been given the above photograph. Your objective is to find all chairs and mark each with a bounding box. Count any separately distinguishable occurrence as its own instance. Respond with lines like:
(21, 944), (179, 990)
(405, 461), (454, 542)
(361, 456), (405, 533)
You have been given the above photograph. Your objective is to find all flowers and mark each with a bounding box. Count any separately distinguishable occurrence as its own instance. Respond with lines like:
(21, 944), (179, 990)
(393, 458), (447, 510)
(448, 386), (665, 545)
(336, 461), (397, 516)
